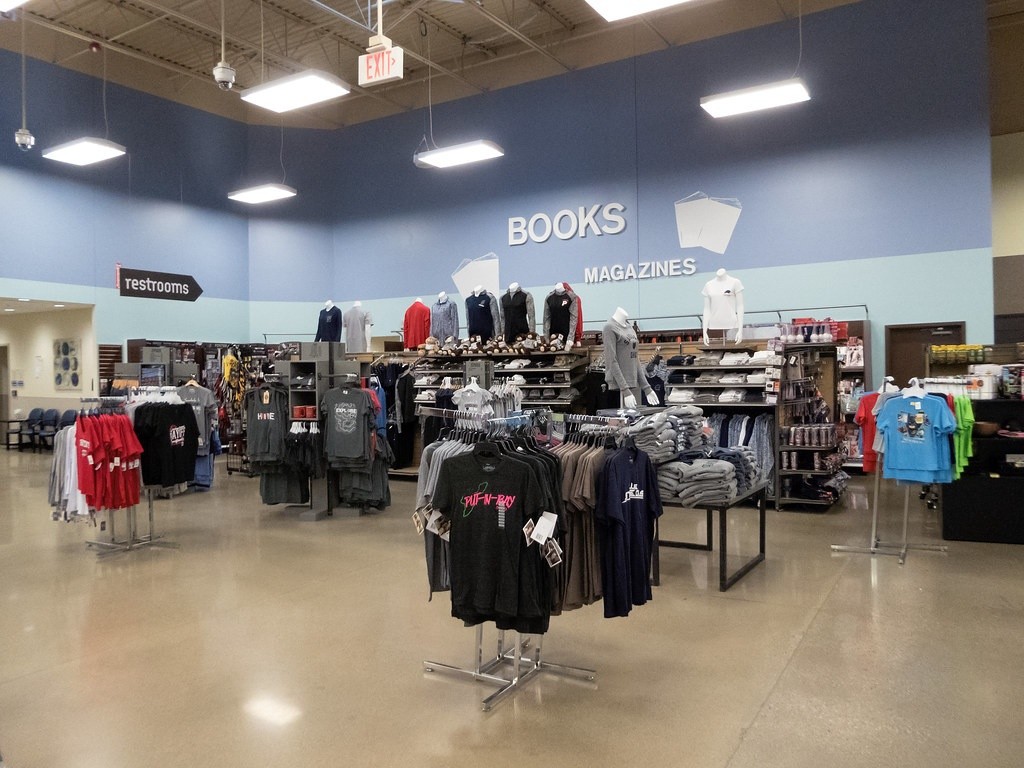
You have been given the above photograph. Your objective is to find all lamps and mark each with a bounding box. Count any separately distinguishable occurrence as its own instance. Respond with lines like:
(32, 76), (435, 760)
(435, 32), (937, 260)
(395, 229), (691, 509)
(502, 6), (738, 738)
(41, 0), (127, 167)
(227, 113), (298, 205)
(418, 3), (505, 169)
(239, 0), (350, 113)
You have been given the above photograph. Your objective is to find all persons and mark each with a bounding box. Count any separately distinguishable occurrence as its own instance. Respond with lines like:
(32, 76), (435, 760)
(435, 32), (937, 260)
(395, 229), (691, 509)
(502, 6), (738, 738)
(314, 300), (342, 342)
(342, 301), (375, 353)
(603, 306), (659, 410)
(403, 282), (583, 352)
(702, 268), (745, 347)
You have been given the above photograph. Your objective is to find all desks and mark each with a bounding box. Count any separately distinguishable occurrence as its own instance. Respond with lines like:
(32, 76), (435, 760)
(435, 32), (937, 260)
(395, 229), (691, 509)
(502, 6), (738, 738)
(0, 418), (28, 450)
(650, 479), (770, 592)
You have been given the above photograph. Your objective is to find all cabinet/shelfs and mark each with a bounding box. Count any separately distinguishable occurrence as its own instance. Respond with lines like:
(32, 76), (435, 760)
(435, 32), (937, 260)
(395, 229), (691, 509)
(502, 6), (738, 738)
(664, 362), (848, 510)
(836, 319), (872, 476)
(923, 341), (1024, 545)
(414, 349), (589, 409)
(288, 360), (330, 420)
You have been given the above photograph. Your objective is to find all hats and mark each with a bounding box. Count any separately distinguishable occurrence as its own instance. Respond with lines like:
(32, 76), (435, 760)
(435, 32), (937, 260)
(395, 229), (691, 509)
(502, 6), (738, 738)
(521, 388), (529, 400)
(540, 388), (557, 399)
(527, 390), (542, 400)
(557, 388), (581, 399)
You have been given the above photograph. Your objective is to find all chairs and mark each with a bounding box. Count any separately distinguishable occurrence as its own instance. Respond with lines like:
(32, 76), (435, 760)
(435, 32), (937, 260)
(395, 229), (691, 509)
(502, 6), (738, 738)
(19, 407), (78, 453)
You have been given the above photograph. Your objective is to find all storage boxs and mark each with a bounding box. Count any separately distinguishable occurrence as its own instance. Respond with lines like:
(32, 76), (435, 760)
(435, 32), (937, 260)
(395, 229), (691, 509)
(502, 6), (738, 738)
(966, 364), (1024, 401)
(836, 345), (864, 367)
(845, 423), (860, 457)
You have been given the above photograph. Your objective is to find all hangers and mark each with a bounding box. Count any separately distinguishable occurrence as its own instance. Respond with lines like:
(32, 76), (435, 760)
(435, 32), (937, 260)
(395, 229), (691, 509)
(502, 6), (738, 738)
(80, 397), (126, 417)
(438, 407), (544, 459)
(127, 386), (185, 404)
(340, 374), (353, 392)
(441, 376), (481, 394)
(562, 416), (638, 455)
(246, 372), (289, 399)
(184, 374), (200, 389)
(492, 376), (515, 389)
(290, 422), (321, 434)
(901, 376), (927, 399)
